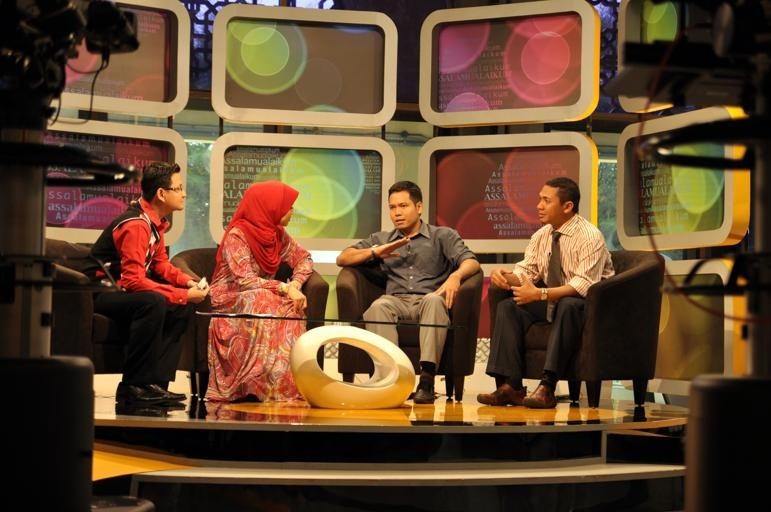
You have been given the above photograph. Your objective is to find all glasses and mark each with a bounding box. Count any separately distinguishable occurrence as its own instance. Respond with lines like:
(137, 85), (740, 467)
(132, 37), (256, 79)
(164, 184), (185, 194)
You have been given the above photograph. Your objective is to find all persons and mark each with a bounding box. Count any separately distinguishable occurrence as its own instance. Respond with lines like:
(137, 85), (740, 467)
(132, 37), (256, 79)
(336, 181), (481, 403)
(80, 161), (210, 409)
(477, 176), (616, 410)
(204, 180), (314, 403)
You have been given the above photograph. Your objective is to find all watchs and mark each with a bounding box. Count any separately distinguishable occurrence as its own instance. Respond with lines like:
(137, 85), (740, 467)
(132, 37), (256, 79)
(542, 288), (548, 301)
(370, 244), (378, 258)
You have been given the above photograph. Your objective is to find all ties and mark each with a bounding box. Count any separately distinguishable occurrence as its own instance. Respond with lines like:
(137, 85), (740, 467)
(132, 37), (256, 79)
(546, 231), (564, 324)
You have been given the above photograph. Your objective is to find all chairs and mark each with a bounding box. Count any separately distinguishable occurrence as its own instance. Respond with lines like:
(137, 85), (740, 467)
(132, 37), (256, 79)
(45, 239), (198, 419)
(170, 247), (330, 420)
(335, 266), (485, 403)
(488, 249), (665, 423)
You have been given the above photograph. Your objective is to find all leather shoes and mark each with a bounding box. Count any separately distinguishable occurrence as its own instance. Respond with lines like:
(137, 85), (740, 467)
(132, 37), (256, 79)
(115, 383), (186, 406)
(414, 381), (435, 405)
(477, 384), (527, 407)
(521, 384), (558, 410)
